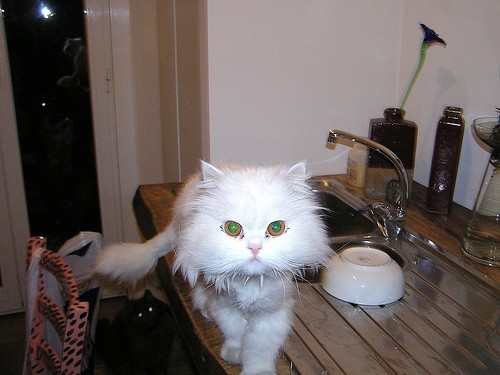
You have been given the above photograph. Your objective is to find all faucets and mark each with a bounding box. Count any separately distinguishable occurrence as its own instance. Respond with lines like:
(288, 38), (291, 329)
(327, 129), (408, 221)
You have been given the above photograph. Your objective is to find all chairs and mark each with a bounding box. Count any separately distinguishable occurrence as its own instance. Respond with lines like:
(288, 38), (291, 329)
(21, 236), (89, 375)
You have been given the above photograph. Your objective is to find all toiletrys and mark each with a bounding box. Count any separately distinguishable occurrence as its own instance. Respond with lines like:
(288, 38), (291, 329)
(424, 106), (465, 216)
(345, 143), (368, 188)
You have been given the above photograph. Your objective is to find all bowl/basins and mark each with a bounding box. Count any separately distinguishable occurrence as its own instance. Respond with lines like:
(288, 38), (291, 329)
(319, 246), (405, 306)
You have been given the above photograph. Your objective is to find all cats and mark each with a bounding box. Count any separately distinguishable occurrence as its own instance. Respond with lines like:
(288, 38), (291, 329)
(93, 159), (352, 375)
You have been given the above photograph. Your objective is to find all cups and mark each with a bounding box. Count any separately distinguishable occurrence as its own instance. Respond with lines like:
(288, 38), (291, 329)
(461, 133), (500, 267)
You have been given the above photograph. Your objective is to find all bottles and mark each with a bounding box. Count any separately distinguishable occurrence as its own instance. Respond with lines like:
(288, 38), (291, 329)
(365, 108), (417, 199)
(425, 107), (465, 215)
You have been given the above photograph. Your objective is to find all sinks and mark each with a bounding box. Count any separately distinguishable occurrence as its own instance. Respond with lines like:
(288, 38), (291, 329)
(292, 181), (409, 284)
